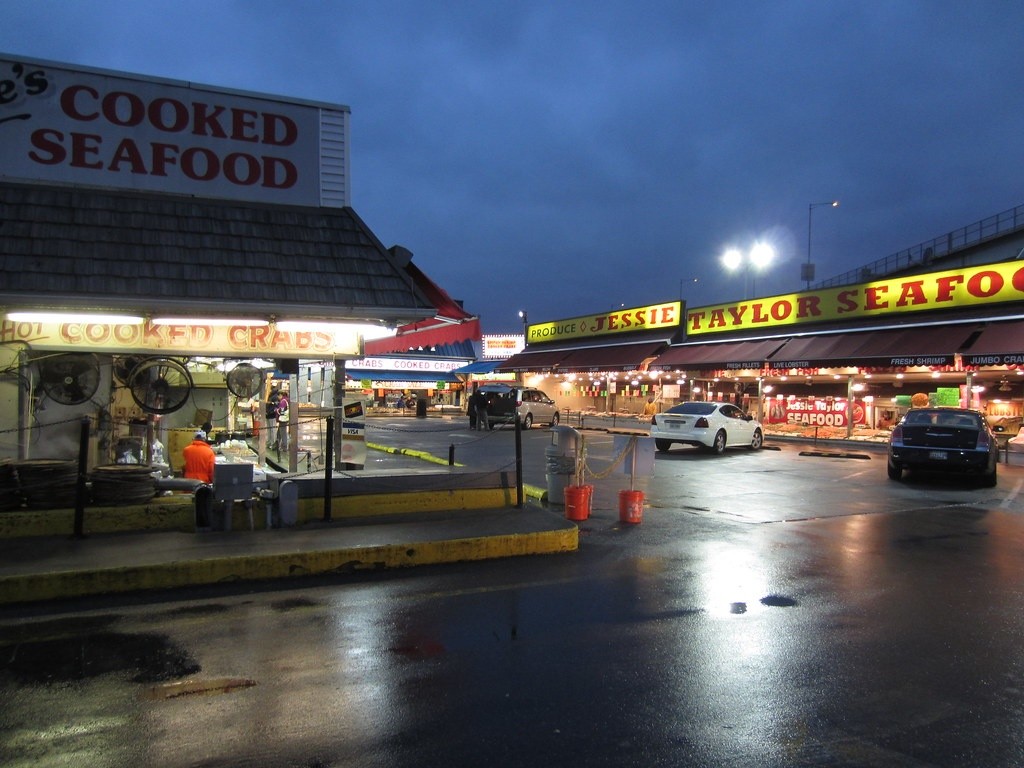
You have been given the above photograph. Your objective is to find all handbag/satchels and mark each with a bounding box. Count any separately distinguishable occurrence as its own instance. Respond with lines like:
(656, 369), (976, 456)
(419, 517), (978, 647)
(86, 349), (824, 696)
(278, 401), (290, 422)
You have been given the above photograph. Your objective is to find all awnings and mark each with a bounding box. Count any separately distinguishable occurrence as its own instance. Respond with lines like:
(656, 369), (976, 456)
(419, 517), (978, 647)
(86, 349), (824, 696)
(359, 289), (482, 357)
(346, 370), (463, 384)
(452, 318), (1024, 374)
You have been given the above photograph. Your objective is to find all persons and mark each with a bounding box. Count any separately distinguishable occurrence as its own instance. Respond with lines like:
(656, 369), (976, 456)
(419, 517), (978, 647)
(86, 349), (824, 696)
(532, 394), (539, 401)
(182, 430), (215, 485)
(643, 398), (656, 414)
(468, 390), (489, 431)
(266, 382), (278, 449)
(195, 422), (215, 444)
(406, 397), (415, 408)
(398, 396), (405, 409)
(272, 391), (290, 452)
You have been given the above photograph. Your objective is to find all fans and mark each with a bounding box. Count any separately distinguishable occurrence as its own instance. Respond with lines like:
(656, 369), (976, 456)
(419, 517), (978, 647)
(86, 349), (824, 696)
(226, 363), (264, 398)
(41, 352), (195, 415)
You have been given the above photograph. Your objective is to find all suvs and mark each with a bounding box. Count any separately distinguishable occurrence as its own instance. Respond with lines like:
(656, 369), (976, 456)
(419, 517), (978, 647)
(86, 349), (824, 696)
(476, 383), (561, 430)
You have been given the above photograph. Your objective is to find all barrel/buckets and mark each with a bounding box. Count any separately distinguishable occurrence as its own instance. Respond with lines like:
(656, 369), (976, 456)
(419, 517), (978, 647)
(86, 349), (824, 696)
(617, 490), (644, 522)
(564, 485), (594, 520)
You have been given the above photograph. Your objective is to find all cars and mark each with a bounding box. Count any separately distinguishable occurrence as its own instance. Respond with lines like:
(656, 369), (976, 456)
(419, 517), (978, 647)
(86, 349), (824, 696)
(651, 402), (764, 455)
(886, 407), (1005, 484)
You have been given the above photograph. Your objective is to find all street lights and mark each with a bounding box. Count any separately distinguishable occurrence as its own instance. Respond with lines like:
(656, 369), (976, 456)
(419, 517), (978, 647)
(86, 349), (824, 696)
(807, 201), (839, 288)
(680, 278), (699, 299)
(518, 311), (528, 348)
(610, 304), (625, 311)
(725, 243), (773, 297)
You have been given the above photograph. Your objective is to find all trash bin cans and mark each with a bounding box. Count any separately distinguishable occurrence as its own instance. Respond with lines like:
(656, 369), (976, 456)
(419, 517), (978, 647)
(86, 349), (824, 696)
(544, 425), (583, 504)
(416, 398), (427, 418)
